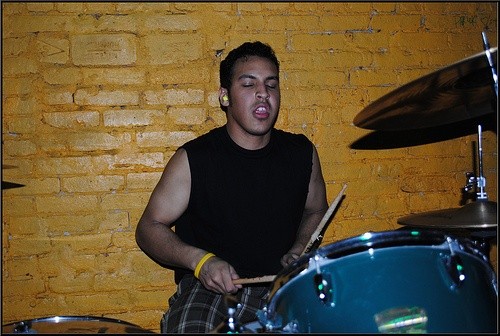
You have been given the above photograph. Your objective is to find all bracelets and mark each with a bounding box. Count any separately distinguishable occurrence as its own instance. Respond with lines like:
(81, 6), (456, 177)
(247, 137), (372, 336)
(194, 252), (215, 278)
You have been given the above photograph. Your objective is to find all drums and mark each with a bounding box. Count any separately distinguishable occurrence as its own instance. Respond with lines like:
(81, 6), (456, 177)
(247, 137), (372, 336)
(226, 230), (498, 336)
(2, 315), (150, 335)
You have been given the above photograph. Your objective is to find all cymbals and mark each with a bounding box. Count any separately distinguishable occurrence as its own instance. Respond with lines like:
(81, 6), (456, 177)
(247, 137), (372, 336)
(396, 200), (498, 227)
(2, 164), (19, 170)
(354, 47), (498, 131)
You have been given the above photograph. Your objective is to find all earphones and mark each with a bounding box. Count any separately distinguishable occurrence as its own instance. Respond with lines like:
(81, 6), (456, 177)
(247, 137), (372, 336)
(222, 95), (228, 101)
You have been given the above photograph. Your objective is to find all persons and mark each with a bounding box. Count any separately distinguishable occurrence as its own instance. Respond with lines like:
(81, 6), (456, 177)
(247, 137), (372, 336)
(135, 41), (329, 336)
